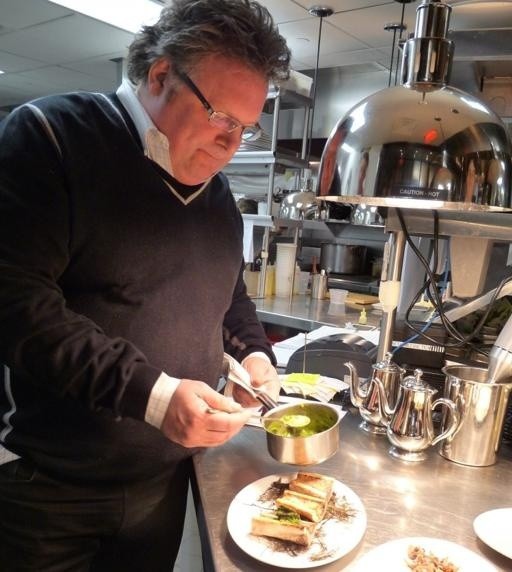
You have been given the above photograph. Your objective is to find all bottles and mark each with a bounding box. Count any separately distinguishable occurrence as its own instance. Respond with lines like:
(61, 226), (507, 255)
(482, 314), (512, 384)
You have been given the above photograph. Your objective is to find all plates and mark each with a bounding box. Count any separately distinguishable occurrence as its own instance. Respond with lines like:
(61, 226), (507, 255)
(473, 507), (511, 560)
(345, 536), (500, 572)
(227, 471), (366, 570)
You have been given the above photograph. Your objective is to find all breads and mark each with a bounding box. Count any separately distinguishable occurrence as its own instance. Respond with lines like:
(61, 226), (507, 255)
(251, 472), (333, 548)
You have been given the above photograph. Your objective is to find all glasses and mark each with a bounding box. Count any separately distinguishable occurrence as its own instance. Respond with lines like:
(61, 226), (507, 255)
(172, 60), (263, 143)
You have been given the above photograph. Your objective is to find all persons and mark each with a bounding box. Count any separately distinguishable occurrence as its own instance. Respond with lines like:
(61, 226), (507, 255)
(1, 1), (296, 572)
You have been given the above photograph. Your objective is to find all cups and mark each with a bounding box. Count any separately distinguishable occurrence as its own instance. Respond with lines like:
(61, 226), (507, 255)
(329, 289), (349, 304)
(327, 303), (346, 317)
(310, 273), (328, 300)
(271, 293), (328, 323)
(295, 271), (310, 295)
(440, 363), (512, 468)
(274, 241), (297, 298)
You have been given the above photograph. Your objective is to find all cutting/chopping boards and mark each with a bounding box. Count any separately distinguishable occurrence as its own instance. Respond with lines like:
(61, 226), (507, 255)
(328, 290), (379, 305)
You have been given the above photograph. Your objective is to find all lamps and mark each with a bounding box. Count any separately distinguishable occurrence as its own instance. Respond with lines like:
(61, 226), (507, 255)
(279, 5), (329, 222)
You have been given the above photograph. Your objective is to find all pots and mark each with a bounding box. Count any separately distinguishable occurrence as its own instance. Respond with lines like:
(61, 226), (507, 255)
(321, 242), (368, 277)
(248, 385), (340, 467)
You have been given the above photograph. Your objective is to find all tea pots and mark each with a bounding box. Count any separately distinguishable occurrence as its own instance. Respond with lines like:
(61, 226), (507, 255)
(342, 352), (406, 436)
(372, 367), (461, 462)
(344, 201), (386, 226)
(274, 177), (322, 222)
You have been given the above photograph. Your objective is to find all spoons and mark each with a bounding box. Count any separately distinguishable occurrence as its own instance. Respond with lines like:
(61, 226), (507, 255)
(206, 408), (312, 431)
